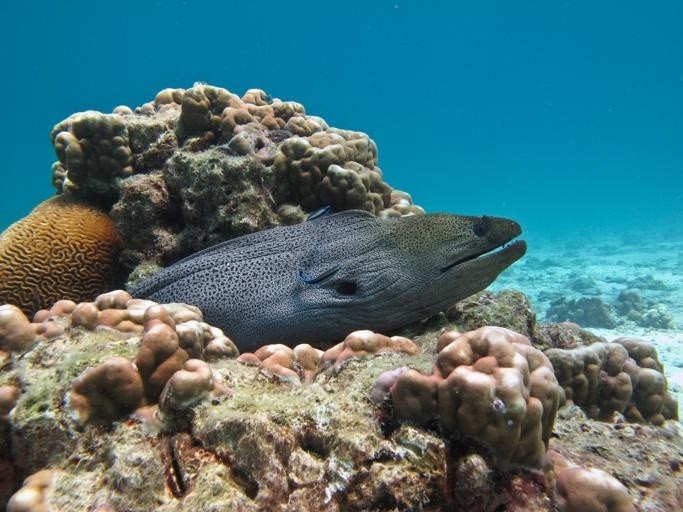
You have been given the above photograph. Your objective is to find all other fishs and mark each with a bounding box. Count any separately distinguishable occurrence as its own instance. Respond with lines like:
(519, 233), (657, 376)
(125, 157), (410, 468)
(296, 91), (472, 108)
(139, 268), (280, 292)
(125, 209), (527, 354)
